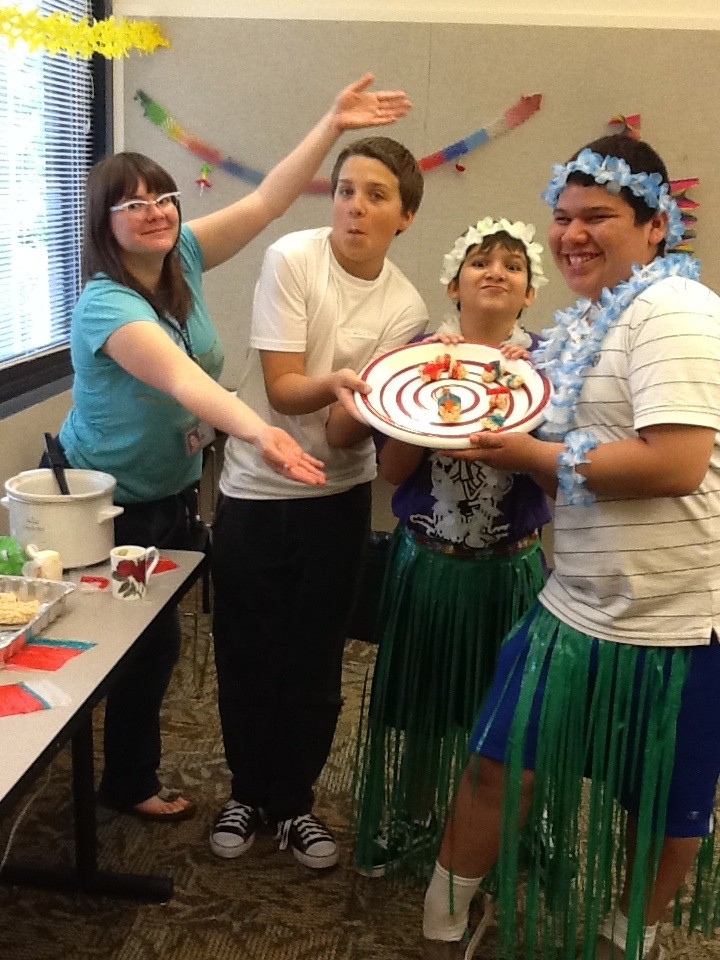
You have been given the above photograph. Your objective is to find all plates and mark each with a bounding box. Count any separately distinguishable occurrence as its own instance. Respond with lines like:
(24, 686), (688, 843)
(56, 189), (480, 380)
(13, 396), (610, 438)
(353, 341), (555, 449)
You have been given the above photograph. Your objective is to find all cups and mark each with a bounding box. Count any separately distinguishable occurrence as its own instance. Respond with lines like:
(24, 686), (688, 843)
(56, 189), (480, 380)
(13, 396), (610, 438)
(110, 546), (159, 601)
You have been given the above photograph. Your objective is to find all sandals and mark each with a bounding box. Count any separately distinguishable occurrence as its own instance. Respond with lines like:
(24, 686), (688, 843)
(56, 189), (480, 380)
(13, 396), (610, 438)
(100, 784), (196, 821)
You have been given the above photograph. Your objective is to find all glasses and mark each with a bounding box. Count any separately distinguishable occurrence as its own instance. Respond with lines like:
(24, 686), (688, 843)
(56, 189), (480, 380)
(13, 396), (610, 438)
(109, 191), (180, 218)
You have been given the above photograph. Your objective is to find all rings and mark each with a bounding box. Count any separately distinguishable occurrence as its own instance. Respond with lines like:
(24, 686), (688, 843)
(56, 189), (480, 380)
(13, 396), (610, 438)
(379, 109), (383, 117)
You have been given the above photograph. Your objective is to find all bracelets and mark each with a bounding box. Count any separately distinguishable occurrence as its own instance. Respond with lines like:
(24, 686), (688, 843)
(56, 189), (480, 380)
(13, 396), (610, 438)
(556, 429), (602, 508)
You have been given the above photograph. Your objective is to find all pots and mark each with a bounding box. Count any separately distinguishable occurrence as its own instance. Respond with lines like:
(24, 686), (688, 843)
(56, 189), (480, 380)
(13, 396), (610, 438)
(0, 469), (125, 571)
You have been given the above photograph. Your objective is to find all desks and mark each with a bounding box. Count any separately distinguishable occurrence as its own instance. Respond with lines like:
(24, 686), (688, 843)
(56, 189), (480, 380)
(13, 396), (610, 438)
(0, 548), (209, 903)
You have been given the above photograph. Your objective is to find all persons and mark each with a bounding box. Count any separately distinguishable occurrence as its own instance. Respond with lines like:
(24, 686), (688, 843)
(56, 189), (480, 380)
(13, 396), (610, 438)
(353, 134), (720, 960)
(541, 496), (542, 499)
(211, 135), (428, 867)
(38, 74), (415, 818)
(189, 432), (200, 452)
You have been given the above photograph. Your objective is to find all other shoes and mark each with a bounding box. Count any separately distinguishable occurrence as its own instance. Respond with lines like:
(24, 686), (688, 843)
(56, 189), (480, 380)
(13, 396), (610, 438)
(577, 931), (662, 960)
(421, 888), (491, 960)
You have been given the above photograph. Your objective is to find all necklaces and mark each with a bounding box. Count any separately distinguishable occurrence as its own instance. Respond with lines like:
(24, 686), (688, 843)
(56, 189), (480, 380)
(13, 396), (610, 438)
(529, 254), (700, 441)
(429, 312), (533, 549)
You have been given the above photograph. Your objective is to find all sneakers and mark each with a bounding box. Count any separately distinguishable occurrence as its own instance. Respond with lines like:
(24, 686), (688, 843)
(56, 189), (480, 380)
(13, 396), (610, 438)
(267, 811), (339, 868)
(355, 810), (438, 875)
(209, 798), (260, 858)
